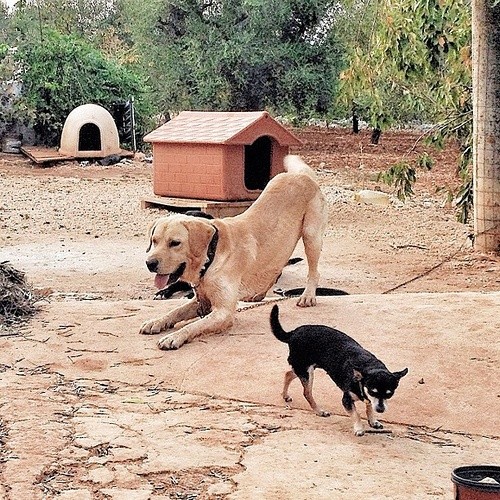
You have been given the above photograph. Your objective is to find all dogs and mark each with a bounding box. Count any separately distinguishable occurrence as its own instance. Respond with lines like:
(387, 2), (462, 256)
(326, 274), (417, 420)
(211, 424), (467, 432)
(268, 303), (410, 439)
(136, 153), (329, 353)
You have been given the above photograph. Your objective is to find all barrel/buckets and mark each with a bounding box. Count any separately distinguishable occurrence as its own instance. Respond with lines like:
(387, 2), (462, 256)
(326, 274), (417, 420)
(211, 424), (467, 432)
(450, 463), (500, 500)
